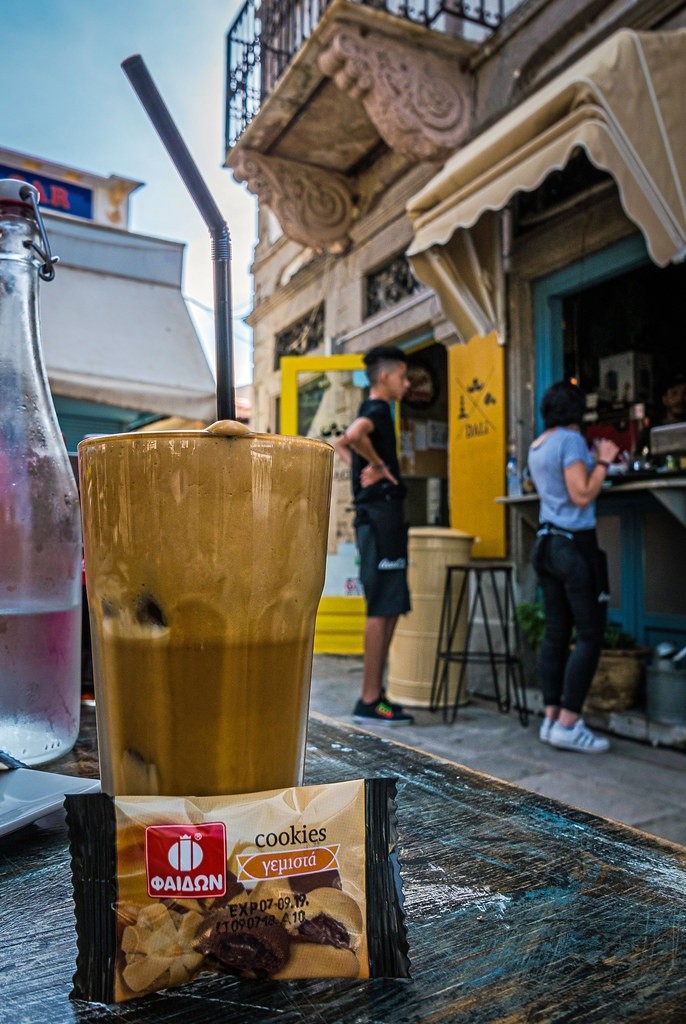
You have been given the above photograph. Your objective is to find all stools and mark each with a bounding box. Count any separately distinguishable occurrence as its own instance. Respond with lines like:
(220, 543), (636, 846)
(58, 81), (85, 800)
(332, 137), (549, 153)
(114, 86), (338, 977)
(427, 560), (532, 727)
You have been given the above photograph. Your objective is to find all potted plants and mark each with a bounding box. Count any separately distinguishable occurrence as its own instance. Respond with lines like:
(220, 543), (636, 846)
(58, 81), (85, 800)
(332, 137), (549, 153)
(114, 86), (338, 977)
(511, 589), (650, 718)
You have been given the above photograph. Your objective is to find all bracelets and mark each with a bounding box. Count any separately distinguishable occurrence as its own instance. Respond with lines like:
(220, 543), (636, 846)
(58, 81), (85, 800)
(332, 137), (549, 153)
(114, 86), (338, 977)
(597, 460), (609, 467)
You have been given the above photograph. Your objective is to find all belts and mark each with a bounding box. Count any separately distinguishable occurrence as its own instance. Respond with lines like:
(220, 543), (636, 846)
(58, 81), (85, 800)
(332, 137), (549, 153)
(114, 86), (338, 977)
(534, 528), (574, 540)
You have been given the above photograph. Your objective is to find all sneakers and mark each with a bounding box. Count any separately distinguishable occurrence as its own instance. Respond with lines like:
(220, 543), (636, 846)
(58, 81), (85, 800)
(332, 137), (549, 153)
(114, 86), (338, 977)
(539, 716), (552, 742)
(549, 717), (610, 754)
(351, 697), (415, 725)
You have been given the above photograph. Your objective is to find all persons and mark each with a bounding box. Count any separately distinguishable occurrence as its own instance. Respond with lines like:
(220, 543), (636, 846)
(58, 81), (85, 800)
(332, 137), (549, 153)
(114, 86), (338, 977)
(520, 380), (621, 752)
(334, 344), (417, 724)
(635, 373), (686, 466)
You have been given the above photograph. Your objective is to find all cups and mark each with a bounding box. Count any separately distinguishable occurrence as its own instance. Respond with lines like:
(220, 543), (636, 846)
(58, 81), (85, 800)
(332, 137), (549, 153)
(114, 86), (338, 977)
(79, 436), (335, 794)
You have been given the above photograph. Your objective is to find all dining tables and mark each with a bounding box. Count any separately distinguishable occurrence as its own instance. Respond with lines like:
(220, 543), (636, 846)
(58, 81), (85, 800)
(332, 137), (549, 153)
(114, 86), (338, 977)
(0, 699), (686, 1024)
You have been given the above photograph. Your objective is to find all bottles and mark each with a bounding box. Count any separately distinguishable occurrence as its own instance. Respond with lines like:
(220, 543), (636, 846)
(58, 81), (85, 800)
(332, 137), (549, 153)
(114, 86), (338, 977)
(0, 179), (82, 768)
(506, 456), (521, 497)
